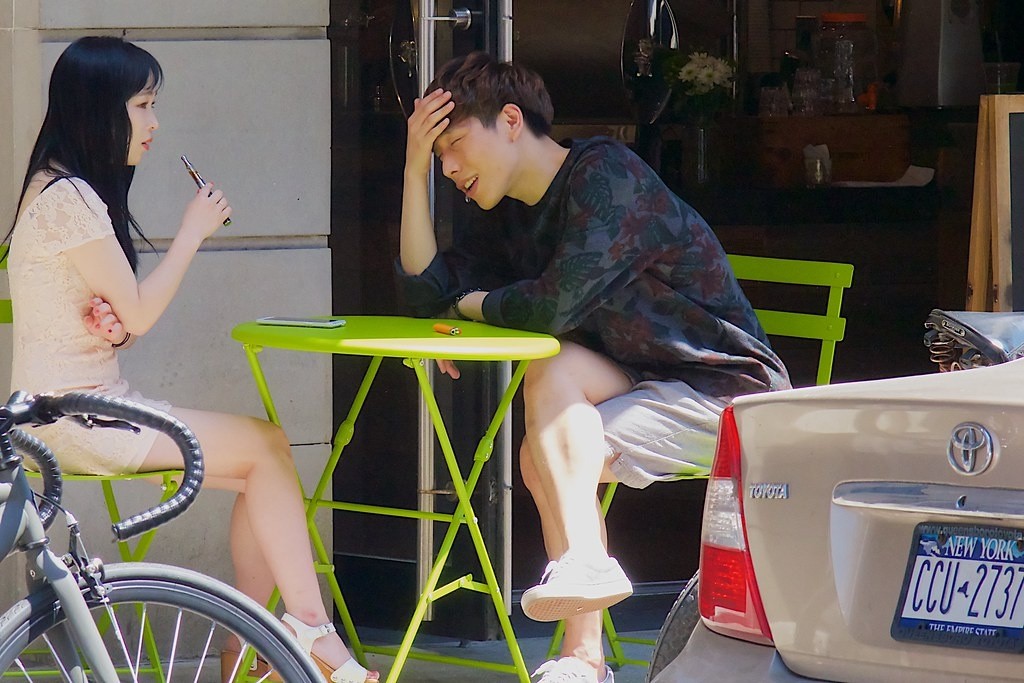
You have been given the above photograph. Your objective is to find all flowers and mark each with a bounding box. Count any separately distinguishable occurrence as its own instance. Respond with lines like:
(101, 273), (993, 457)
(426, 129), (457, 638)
(634, 37), (662, 79)
(662, 51), (736, 172)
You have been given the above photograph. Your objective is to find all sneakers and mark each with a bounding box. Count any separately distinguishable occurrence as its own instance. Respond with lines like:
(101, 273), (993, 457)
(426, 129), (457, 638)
(520, 545), (633, 622)
(530, 655), (615, 683)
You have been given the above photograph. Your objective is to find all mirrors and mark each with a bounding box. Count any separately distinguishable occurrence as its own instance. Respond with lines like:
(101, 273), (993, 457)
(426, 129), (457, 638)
(620, 0), (681, 124)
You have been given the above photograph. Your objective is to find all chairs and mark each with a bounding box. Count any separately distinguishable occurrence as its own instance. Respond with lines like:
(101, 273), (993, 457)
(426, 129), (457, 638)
(0, 242), (186, 683)
(548, 254), (854, 666)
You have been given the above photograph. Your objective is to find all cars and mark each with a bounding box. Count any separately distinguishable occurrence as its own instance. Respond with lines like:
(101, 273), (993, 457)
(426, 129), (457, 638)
(646, 355), (1024, 682)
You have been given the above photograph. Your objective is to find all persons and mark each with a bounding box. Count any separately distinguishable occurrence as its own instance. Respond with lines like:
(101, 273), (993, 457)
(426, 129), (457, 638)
(390, 51), (792, 682)
(6, 37), (380, 683)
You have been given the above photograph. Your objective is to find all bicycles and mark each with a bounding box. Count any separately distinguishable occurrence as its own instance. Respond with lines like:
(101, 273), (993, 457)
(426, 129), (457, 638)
(0, 390), (326, 683)
(645, 307), (1023, 682)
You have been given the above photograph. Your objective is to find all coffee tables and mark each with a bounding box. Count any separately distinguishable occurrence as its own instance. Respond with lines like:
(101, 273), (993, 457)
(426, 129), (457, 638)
(230, 315), (561, 683)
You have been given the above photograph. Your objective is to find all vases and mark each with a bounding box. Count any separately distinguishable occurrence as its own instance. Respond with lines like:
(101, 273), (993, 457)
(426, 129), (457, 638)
(683, 116), (726, 190)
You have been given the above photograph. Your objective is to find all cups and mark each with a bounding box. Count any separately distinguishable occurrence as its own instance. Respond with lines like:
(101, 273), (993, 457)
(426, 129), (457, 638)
(791, 67), (822, 121)
(757, 86), (789, 122)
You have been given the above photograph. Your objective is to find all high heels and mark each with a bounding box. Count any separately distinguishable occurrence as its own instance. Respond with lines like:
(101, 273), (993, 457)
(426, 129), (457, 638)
(268, 611), (379, 683)
(220, 633), (283, 683)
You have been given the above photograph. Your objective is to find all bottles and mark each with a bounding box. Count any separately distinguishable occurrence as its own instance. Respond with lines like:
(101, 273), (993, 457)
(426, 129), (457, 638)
(815, 12), (882, 117)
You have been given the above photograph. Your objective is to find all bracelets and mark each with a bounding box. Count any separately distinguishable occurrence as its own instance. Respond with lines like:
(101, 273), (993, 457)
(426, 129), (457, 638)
(453, 288), (482, 322)
(112, 332), (130, 348)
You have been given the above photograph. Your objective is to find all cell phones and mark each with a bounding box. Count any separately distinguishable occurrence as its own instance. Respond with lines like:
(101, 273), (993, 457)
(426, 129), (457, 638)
(256, 316), (346, 328)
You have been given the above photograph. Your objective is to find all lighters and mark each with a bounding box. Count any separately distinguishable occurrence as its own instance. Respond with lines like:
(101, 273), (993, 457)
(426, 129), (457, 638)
(434, 323), (462, 336)
